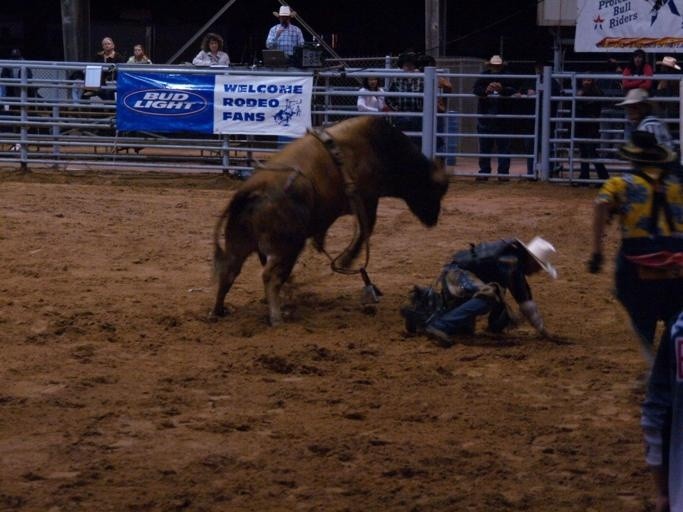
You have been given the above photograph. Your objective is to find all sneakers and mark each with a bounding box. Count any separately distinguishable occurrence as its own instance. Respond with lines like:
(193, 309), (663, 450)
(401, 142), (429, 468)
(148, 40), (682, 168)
(400, 305), (448, 346)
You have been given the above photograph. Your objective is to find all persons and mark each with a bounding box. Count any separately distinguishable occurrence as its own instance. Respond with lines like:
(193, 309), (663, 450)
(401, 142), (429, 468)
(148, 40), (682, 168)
(191, 32), (230, 68)
(0, 48), (34, 154)
(80, 36), (126, 120)
(357, 49), (682, 188)
(264, 4), (305, 62)
(125, 43), (153, 65)
(580, 128), (682, 354)
(640, 310), (681, 512)
(401, 232), (574, 348)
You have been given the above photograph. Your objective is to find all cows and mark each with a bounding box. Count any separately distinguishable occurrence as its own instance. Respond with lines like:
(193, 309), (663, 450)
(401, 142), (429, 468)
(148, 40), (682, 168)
(204, 112), (451, 329)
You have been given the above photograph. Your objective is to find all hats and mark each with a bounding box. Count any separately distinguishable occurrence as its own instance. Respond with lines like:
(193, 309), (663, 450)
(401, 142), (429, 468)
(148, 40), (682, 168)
(486, 55), (504, 65)
(617, 131), (676, 163)
(615, 89), (659, 111)
(517, 237), (557, 278)
(273, 6), (297, 18)
(656, 56), (681, 70)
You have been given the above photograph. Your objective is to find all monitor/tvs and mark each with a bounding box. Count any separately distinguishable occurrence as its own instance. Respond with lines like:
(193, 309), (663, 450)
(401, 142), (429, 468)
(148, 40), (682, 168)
(301, 47), (324, 68)
(262, 50), (284, 64)
